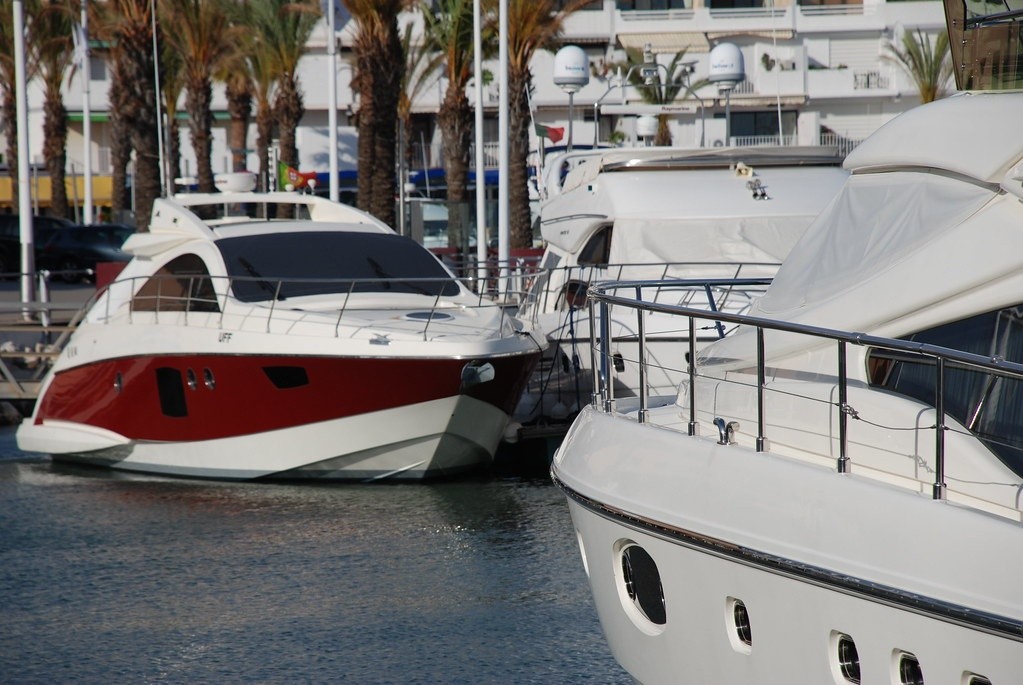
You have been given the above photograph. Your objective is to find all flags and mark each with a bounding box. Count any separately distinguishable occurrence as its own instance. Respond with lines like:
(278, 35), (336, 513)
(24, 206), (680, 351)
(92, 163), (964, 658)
(279, 159), (319, 191)
(535, 122), (564, 143)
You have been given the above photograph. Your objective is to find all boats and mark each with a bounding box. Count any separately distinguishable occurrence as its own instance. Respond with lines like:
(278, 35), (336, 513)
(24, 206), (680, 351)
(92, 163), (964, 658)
(14, 175), (549, 484)
(514, 43), (1023, 684)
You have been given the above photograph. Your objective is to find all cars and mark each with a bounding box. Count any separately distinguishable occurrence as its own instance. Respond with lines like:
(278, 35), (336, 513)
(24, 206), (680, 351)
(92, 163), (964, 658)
(43, 225), (137, 288)
(397, 196), (449, 248)
(0, 214), (75, 279)
